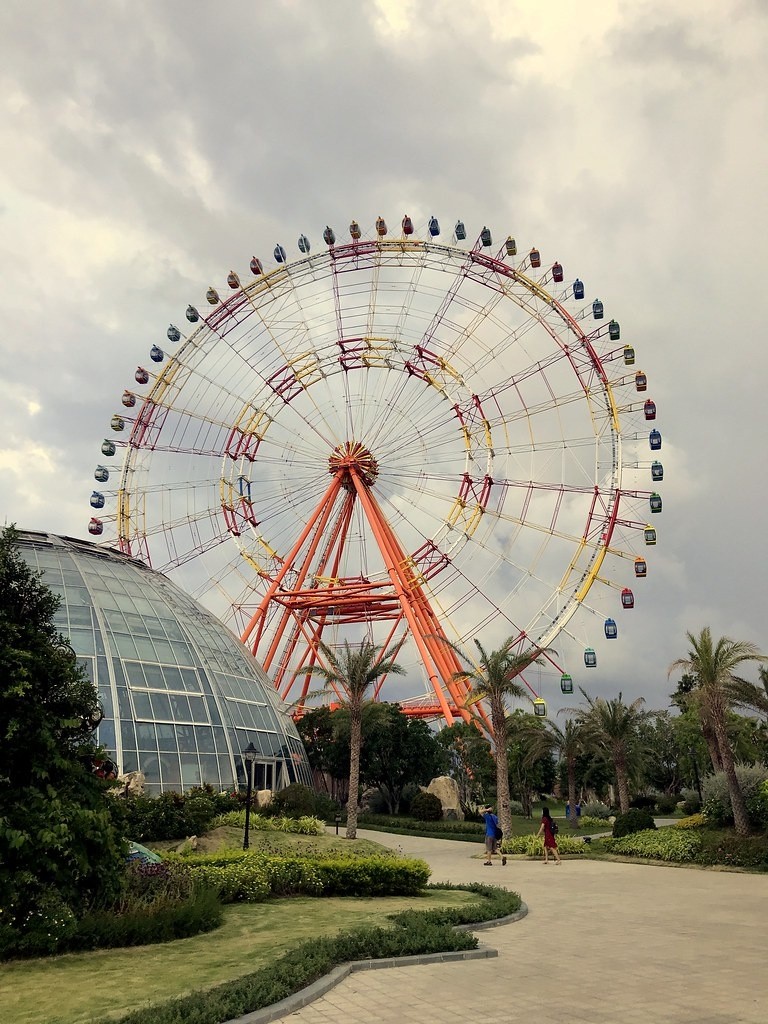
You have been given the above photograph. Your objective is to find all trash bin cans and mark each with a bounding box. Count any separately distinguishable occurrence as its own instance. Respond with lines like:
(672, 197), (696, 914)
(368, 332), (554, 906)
(566, 805), (581, 818)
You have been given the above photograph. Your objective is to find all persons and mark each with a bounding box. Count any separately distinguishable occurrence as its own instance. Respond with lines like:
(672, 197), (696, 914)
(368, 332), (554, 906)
(479, 805), (507, 866)
(536, 807), (562, 865)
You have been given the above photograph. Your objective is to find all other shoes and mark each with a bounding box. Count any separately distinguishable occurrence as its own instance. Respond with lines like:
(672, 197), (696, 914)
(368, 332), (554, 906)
(484, 862), (492, 865)
(502, 857), (506, 865)
(543, 862), (548, 864)
(555, 860), (561, 865)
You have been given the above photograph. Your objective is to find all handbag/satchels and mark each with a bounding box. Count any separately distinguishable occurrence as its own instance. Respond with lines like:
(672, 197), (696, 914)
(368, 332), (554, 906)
(550, 822), (555, 834)
(495, 828), (503, 840)
